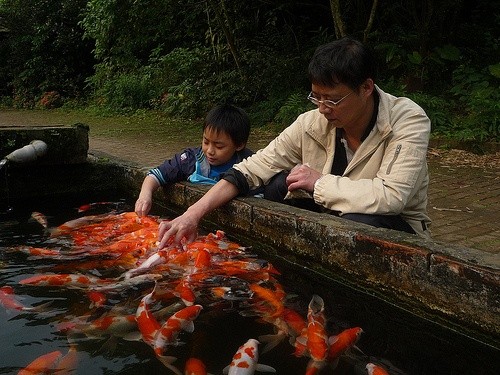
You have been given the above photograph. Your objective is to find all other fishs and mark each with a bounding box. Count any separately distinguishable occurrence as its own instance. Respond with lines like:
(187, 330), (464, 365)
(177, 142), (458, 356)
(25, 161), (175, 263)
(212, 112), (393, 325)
(0, 200), (391, 375)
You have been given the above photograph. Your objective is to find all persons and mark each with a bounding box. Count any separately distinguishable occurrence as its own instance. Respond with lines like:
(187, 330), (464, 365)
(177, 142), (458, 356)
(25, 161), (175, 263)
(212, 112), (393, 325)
(155, 37), (433, 253)
(134, 103), (288, 218)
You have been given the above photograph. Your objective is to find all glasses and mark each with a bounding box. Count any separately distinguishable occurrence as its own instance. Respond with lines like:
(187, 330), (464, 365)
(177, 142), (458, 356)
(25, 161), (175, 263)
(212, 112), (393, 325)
(306, 91), (353, 109)
